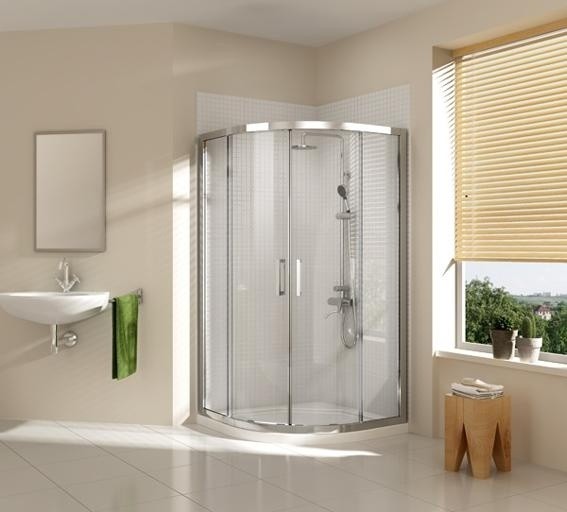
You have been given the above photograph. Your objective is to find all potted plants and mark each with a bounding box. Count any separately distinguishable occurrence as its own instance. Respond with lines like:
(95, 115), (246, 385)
(489, 308), (543, 363)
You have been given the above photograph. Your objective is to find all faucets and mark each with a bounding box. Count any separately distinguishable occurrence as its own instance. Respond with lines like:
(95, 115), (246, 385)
(59, 256), (69, 286)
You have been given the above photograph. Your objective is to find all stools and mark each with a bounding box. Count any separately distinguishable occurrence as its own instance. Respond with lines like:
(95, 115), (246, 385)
(444, 393), (512, 480)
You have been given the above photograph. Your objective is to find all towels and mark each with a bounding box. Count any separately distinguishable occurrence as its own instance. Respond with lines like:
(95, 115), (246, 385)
(112, 293), (139, 381)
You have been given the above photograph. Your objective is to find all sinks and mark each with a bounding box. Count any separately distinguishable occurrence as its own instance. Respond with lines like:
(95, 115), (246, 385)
(0, 293), (111, 323)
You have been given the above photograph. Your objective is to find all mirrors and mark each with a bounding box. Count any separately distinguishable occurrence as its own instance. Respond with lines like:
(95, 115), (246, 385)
(32, 129), (107, 253)
